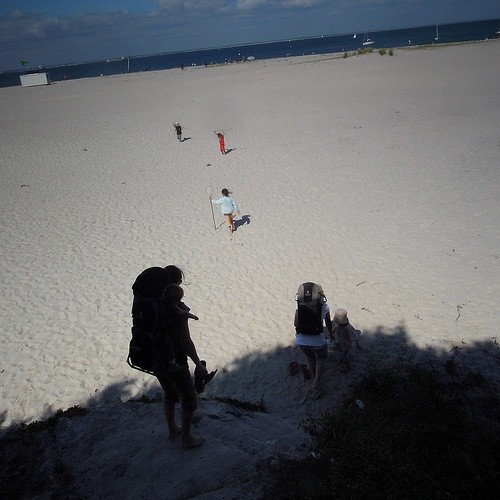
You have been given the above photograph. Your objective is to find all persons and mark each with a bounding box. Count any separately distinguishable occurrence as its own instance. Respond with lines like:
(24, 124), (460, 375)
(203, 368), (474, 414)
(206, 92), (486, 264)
(214, 130), (226, 155)
(181, 64), (184, 71)
(211, 188), (241, 234)
(332, 308), (362, 373)
(174, 122), (184, 142)
(293, 285), (336, 390)
(143, 265), (208, 448)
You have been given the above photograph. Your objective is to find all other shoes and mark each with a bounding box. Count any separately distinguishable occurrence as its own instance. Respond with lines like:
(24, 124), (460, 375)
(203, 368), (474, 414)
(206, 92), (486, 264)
(194, 361), (217, 393)
(301, 364), (311, 380)
(287, 361), (299, 376)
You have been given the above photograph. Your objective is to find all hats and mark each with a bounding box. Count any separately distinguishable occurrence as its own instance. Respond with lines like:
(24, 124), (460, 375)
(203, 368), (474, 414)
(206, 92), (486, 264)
(335, 308), (348, 324)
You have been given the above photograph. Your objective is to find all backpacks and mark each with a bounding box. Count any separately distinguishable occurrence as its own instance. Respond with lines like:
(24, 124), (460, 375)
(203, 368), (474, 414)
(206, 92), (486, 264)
(128, 295), (168, 375)
(294, 283), (324, 335)
